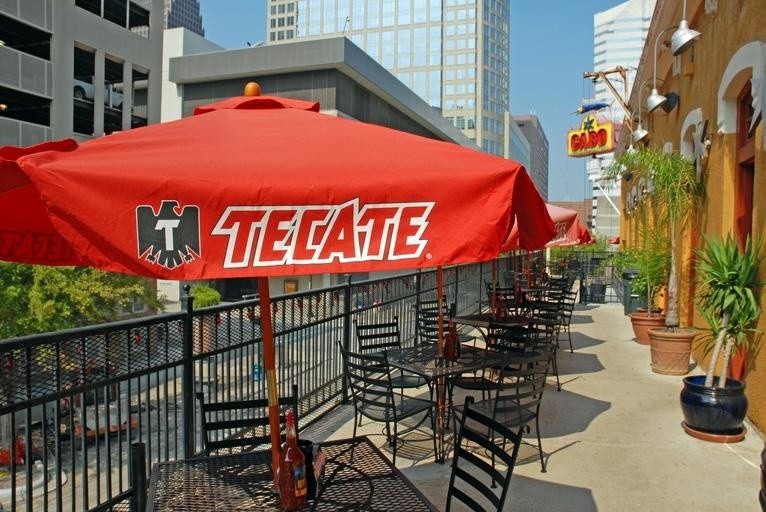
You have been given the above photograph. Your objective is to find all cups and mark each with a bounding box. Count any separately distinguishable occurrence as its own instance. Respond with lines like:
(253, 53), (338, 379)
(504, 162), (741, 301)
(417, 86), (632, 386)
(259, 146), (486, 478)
(284, 440), (317, 500)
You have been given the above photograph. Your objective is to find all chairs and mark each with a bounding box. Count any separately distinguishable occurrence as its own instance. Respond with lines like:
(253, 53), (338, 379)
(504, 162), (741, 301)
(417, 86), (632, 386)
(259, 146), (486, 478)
(450, 343), (558, 489)
(413, 263), (595, 401)
(196, 384), (303, 455)
(351, 315), (436, 436)
(336, 337), (439, 465)
(447, 394), (532, 511)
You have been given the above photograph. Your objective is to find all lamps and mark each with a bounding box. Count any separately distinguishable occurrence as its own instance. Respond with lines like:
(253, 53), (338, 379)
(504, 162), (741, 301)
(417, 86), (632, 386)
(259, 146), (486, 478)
(626, 0), (702, 154)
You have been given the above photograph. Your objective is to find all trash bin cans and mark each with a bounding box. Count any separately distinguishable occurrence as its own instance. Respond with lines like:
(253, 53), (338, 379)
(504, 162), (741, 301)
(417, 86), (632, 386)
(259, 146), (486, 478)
(623, 270), (643, 315)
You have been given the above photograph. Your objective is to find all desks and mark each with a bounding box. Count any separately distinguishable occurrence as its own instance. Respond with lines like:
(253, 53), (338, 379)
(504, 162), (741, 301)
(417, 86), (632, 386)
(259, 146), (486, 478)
(144, 433), (440, 512)
(362, 339), (520, 465)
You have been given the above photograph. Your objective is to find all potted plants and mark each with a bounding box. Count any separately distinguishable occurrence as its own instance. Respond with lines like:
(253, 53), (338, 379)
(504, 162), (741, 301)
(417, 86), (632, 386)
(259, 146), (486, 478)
(678, 227), (764, 434)
(597, 142), (715, 375)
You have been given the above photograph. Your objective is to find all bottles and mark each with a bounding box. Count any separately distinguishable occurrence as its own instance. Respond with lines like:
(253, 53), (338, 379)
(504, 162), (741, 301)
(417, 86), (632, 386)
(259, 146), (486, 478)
(444, 317), (461, 362)
(496, 294), (507, 321)
(278, 409), (308, 512)
(516, 284), (528, 307)
